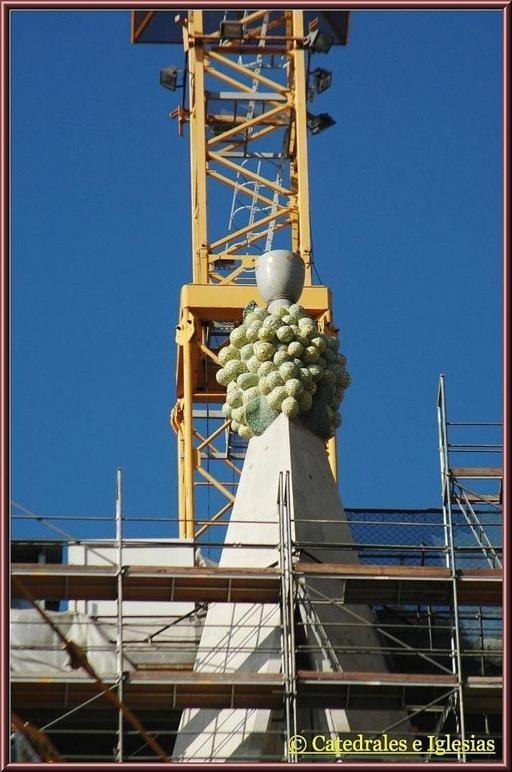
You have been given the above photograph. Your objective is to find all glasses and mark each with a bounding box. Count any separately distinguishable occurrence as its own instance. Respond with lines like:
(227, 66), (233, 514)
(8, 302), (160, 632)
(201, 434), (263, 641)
(159, 66), (181, 91)
(307, 30), (337, 135)
(217, 20), (245, 40)
